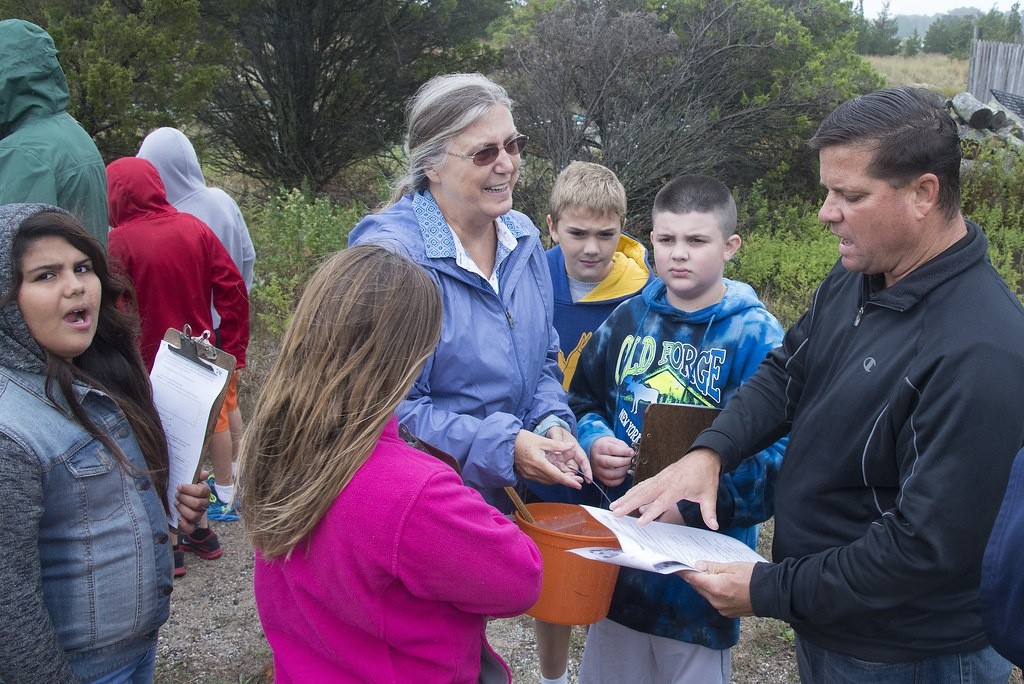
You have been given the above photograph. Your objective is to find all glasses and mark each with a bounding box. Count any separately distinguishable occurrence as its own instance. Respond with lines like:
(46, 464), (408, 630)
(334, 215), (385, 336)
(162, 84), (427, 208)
(444, 133), (530, 166)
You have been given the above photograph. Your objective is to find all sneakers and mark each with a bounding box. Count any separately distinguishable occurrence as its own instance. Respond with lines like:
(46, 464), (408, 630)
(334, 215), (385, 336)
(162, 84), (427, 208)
(204, 473), (242, 521)
(177, 525), (223, 559)
(174, 549), (186, 575)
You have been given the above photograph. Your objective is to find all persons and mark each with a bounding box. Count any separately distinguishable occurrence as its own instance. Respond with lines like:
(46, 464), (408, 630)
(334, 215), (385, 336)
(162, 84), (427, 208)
(136, 126), (256, 523)
(1, 201), (174, 684)
(1, 19), (110, 249)
(535, 160), (656, 684)
(228, 244), (545, 684)
(567, 173), (792, 684)
(609, 85), (1023, 684)
(346, 72), (593, 516)
(106, 157), (250, 579)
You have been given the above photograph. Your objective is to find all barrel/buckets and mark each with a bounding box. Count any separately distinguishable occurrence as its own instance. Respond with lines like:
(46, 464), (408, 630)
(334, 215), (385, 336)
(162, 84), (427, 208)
(516, 462), (621, 627)
